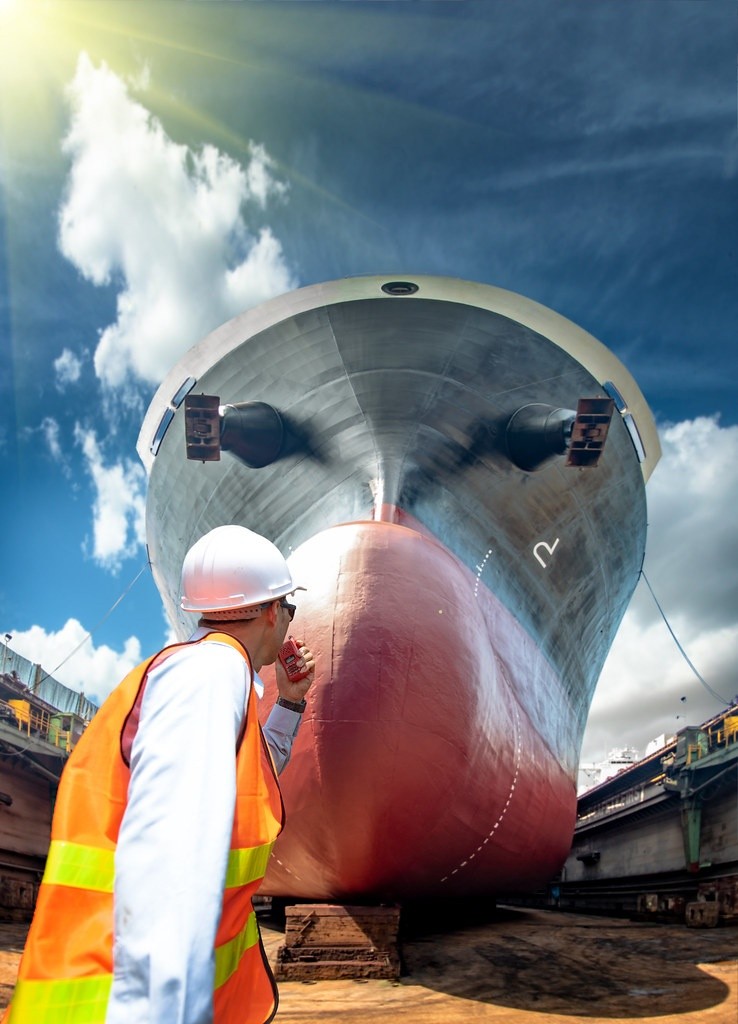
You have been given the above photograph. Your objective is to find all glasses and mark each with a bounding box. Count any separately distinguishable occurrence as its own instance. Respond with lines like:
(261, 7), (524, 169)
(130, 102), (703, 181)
(261, 602), (296, 622)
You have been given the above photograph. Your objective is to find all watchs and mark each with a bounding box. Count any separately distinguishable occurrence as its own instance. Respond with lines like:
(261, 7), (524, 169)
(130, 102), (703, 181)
(276, 696), (308, 714)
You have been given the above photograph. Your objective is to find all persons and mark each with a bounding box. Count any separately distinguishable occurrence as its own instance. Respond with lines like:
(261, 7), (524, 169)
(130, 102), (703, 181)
(8, 526), (317, 1024)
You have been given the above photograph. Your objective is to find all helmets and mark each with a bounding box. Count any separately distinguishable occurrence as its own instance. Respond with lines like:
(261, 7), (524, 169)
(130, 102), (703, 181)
(179, 522), (307, 613)
